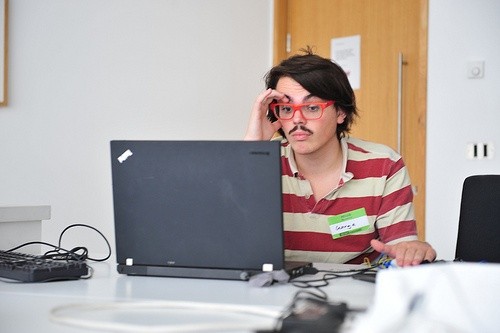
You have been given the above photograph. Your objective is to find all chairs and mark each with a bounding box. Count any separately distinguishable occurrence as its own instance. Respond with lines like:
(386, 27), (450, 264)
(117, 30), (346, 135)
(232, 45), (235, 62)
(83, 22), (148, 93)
(452, 174), (500, 263)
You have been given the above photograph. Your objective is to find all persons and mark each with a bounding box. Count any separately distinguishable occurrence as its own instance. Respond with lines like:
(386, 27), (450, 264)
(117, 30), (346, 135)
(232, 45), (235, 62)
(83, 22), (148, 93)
(244, 54), (437, 268)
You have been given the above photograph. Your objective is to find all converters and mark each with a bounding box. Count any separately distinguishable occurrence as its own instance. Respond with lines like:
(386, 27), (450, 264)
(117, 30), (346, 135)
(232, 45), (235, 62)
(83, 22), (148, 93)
(281, 299), (347, 333)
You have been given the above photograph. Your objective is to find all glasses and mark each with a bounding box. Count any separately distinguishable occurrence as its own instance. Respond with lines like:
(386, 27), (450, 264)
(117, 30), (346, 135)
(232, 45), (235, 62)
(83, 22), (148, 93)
(269, 100), (336, 120)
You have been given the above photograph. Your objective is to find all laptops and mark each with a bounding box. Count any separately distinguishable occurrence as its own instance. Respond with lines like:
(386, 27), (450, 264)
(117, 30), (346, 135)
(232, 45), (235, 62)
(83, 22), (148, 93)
(110, 140), (312, 282)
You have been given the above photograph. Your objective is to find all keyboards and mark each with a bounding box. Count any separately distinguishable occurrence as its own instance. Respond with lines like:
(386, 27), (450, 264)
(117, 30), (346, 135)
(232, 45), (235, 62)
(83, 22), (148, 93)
(0, 249), (90, 283)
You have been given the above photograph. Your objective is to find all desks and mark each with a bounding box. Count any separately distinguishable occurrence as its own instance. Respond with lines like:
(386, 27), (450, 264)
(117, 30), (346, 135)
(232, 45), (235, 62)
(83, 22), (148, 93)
(0, 204), (51, 256)
(0, 261), (500, 333)
